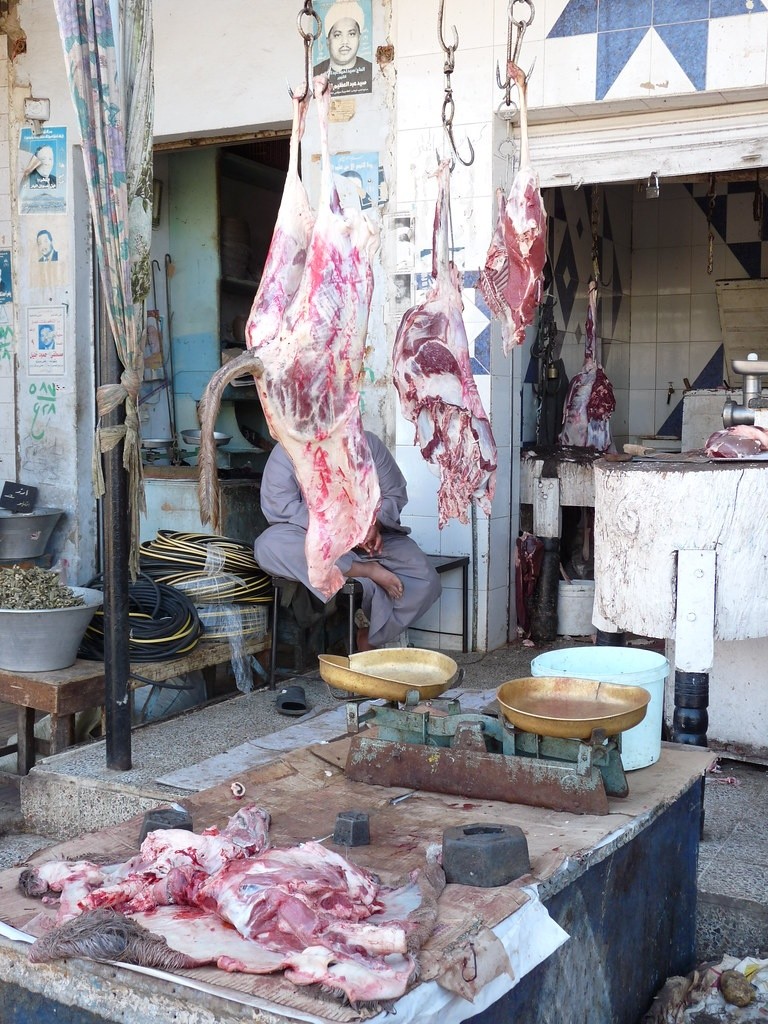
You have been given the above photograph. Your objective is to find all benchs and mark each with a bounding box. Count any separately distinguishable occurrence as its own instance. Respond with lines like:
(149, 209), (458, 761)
(0, 632), (272, 776)
(268, 553), (471, 699)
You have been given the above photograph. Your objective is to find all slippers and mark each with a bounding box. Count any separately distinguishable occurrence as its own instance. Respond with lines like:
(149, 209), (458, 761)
(276, 686), (307, 715)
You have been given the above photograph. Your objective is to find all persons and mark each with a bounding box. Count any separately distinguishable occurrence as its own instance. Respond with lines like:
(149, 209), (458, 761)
(253, 430), (445, 650)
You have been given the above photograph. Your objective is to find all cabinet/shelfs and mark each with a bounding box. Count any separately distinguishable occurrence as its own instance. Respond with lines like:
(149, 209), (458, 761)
(168, 146), (289, 475)
